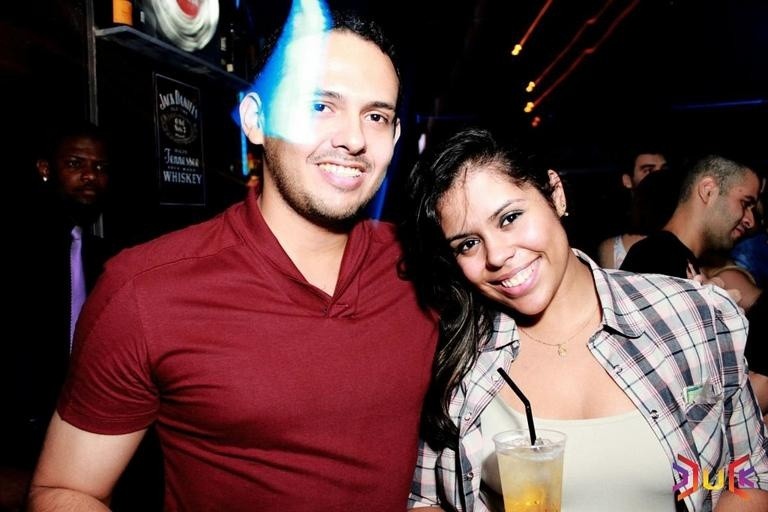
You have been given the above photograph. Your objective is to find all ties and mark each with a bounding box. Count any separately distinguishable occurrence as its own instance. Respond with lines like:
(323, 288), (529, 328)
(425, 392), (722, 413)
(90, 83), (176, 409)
(70, 225), (87, 355)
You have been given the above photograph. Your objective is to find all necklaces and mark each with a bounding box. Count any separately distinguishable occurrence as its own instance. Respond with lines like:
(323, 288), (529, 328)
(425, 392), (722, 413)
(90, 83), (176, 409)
(518, 300), (600, 357)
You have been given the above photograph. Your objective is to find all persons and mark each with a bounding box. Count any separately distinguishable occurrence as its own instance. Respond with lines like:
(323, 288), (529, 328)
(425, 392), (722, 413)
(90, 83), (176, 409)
(26, 9), (442, 511)
(595, 146), (767, 430)
(0, 125), (108, 512)
(397, 129), (768, 512)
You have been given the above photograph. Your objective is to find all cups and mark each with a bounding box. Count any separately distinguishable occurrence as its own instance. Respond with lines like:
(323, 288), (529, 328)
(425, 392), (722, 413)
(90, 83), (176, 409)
(493, 430), (566, 511)
